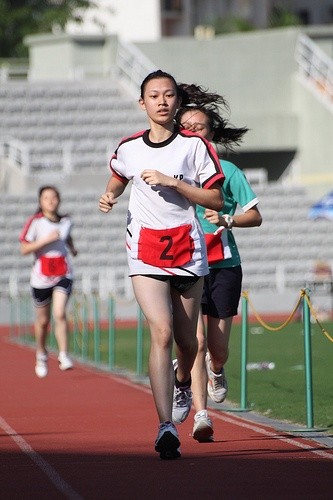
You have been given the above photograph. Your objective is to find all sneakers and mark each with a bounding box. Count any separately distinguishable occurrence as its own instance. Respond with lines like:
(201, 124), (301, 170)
(192, 410), (214, 442)
(171, 359), (193, 423)
(155, 421), (182, 460)
(206, 352), (227, 403)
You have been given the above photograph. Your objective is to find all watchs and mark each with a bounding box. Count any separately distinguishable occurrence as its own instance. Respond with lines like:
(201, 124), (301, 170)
(222, 214), (233, 229)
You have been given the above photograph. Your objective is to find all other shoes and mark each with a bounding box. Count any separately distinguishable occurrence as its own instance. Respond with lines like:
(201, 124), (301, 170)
(35, 350), (49, 378)
(59, 358), (74, 370)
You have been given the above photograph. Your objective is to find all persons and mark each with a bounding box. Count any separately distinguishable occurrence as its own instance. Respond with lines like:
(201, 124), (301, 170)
(176, 105), (262, 443)
(19, 186), (78, 379)
(98, 70), (226, 462)
